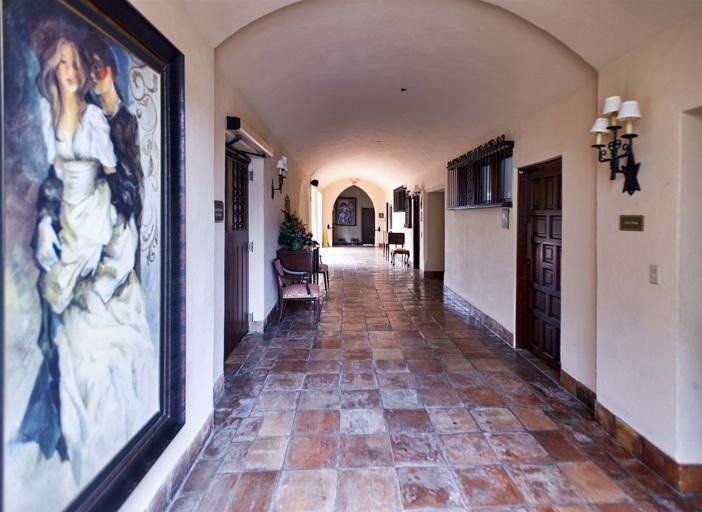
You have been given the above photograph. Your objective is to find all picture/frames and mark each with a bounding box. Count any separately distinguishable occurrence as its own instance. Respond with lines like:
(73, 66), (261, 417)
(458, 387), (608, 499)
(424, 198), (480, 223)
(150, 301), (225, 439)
(0, 1), (186, 512)
(404, 196), (412, 229)
(335, 197), (356, 226)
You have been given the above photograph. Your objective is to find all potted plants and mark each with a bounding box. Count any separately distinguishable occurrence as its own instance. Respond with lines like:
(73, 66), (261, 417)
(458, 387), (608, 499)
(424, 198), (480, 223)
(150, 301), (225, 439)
(277, 211), (315, 250)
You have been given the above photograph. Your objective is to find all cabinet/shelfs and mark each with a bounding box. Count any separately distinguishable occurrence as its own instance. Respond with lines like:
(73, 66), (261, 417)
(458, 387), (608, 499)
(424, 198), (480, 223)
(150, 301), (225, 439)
(277, 246), (320, 313)
(383, 231), (405, 261)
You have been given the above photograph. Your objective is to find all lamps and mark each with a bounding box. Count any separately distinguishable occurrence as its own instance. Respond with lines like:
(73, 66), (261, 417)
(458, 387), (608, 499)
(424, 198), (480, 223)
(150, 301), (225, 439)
(588, 93), (644, 186)
(272, 153), (289, 198)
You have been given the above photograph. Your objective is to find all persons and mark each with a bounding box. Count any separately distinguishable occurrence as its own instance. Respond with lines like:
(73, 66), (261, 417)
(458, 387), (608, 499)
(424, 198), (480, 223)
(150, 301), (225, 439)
(14, 40), (148, 455)
(19, 17), (161, 492)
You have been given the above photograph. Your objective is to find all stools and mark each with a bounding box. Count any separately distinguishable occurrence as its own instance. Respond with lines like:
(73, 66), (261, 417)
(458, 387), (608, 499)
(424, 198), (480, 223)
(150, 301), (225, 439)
(336, 238), (359, 245)
(391, 248), (410, 265)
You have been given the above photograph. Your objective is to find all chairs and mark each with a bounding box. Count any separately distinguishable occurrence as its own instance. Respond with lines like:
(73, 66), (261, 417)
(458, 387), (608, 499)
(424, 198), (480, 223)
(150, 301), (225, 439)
(272, 258), (321, 328)
